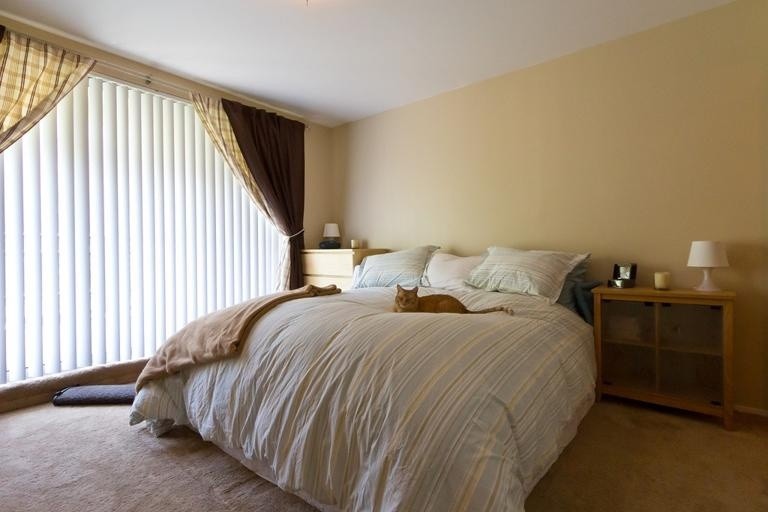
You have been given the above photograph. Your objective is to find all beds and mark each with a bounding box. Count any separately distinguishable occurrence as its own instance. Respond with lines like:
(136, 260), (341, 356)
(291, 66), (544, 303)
(136, 282), (597, 509)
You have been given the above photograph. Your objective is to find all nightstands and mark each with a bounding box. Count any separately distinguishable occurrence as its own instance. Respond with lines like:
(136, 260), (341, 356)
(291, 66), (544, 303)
(303, 247), (389, 291)
(591, 285), (738, 431)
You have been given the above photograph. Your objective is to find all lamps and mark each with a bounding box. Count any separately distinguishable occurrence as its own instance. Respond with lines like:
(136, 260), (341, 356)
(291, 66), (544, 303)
(685, 237), (732, 292)
(321, 223), (341, 248)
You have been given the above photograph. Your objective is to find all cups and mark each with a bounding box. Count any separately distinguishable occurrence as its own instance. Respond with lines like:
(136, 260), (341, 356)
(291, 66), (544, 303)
(351, 240), (359, 249)
(653, 271), (671, 289)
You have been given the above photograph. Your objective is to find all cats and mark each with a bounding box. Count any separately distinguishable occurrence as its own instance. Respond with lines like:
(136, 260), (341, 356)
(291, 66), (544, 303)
(392, 284), (514, 317)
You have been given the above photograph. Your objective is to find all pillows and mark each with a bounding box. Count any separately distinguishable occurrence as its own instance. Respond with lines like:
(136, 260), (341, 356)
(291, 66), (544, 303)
(420, 244), (488, 291)
(463, 242), (594, 308)
(557, 255), (591, 319)
(348, 242), (439, 289)
